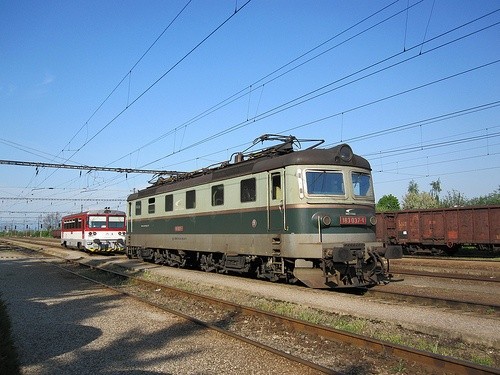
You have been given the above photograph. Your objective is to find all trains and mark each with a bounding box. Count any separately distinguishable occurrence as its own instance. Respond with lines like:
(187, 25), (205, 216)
(377, 204), (500, 255)
(122, 134), (405, 292)
(58, 206), (125, 255)
